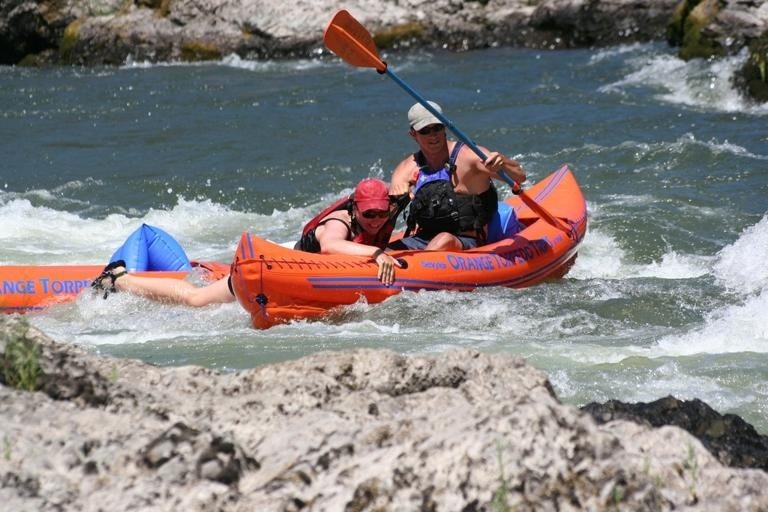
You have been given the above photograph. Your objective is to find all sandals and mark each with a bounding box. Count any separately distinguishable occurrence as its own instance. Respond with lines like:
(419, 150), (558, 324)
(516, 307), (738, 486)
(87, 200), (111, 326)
(91, 260), (128, 300)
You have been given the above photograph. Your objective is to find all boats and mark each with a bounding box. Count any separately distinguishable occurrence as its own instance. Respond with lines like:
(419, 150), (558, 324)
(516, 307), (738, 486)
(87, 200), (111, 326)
(229, 164), (585, 330)
(0, 262), (232, 315)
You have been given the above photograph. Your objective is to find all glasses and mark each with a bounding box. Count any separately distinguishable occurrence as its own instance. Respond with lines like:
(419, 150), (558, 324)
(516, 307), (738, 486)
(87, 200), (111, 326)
(362, 210), (389, 219)
(418, 125), (442, 137)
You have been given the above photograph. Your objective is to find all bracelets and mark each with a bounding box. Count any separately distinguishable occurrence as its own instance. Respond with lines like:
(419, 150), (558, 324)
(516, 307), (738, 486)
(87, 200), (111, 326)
(372, 249), (382, 259)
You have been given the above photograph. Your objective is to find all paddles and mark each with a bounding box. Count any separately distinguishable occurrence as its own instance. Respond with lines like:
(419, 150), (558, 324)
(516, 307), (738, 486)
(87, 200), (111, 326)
(323, 10), (572, 235)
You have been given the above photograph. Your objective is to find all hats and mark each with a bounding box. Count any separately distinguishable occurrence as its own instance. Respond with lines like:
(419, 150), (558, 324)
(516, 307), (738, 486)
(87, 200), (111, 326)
(408, 100), (444, 132)
(353, 178), (390, 213)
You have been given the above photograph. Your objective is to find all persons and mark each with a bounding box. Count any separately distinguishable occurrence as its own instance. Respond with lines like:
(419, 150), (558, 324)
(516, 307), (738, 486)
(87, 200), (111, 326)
(91, 178), (402, 307)
(387, 100), (526, 251)
